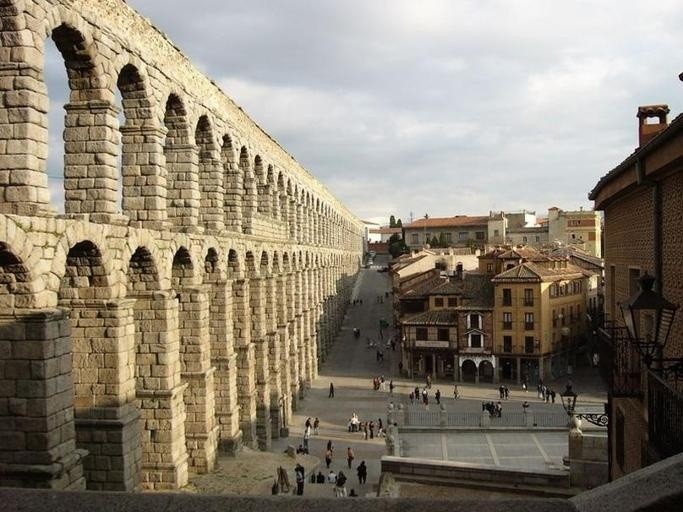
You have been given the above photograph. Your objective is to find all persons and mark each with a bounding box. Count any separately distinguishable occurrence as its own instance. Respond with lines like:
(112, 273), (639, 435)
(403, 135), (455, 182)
(582, 350), (602, 369)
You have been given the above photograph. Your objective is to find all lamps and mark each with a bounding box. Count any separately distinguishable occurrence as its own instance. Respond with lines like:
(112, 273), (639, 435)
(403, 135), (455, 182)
(617, 268), (680, 381)
(560, 384), (609, 429)
